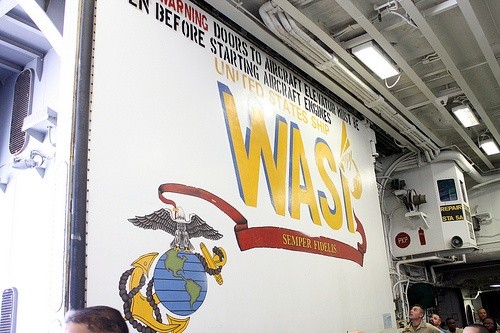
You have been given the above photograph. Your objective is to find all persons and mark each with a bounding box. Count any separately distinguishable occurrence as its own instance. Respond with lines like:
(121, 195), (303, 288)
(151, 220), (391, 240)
(60, 306), (130, 333)
(400, 304), (500, 333)
